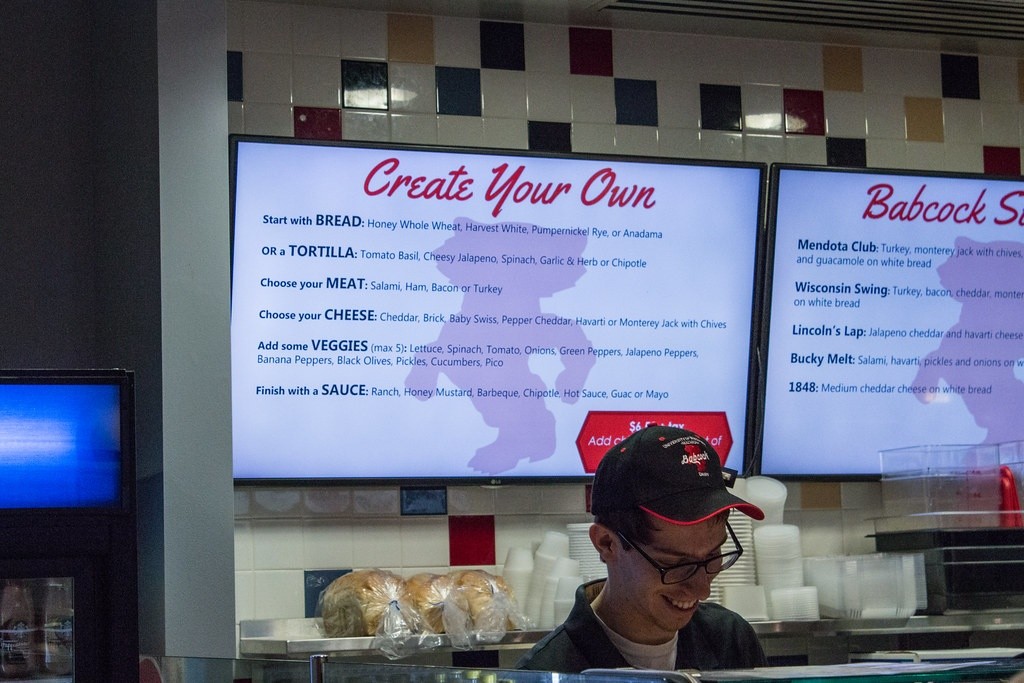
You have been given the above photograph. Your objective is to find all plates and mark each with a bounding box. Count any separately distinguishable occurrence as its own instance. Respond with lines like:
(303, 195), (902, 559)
(699, 504), (758, 609)
(568, 523), (608, 583)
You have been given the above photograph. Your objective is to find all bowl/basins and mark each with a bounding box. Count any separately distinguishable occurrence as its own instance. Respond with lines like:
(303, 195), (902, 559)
(502, 531), (579, 630)
(724, 477), (820, 622)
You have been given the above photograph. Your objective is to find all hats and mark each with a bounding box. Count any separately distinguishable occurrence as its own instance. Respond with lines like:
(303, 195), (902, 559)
(591, 421), (765, 525)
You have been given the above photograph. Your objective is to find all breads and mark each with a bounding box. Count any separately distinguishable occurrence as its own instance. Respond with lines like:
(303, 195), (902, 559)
(321, 569), (518, 636)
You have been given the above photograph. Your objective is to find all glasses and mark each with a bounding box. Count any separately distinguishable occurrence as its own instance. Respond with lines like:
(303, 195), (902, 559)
(614, 522), (742, 584)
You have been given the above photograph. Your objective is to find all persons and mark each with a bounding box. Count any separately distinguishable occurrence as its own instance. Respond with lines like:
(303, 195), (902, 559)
(513, 427), (768, 673)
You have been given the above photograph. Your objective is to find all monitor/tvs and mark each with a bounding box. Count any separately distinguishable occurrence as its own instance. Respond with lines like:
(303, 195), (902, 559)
(225, 133), (769, 490)
(0, 376), (128, 516)
(756, 160), (1024, 483)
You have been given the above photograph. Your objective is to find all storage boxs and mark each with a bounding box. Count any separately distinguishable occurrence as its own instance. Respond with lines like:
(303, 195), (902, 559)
(865, 528), (1024, 616)
(879, 440), (1024, 529)
(848, 648), (1024, 664)
